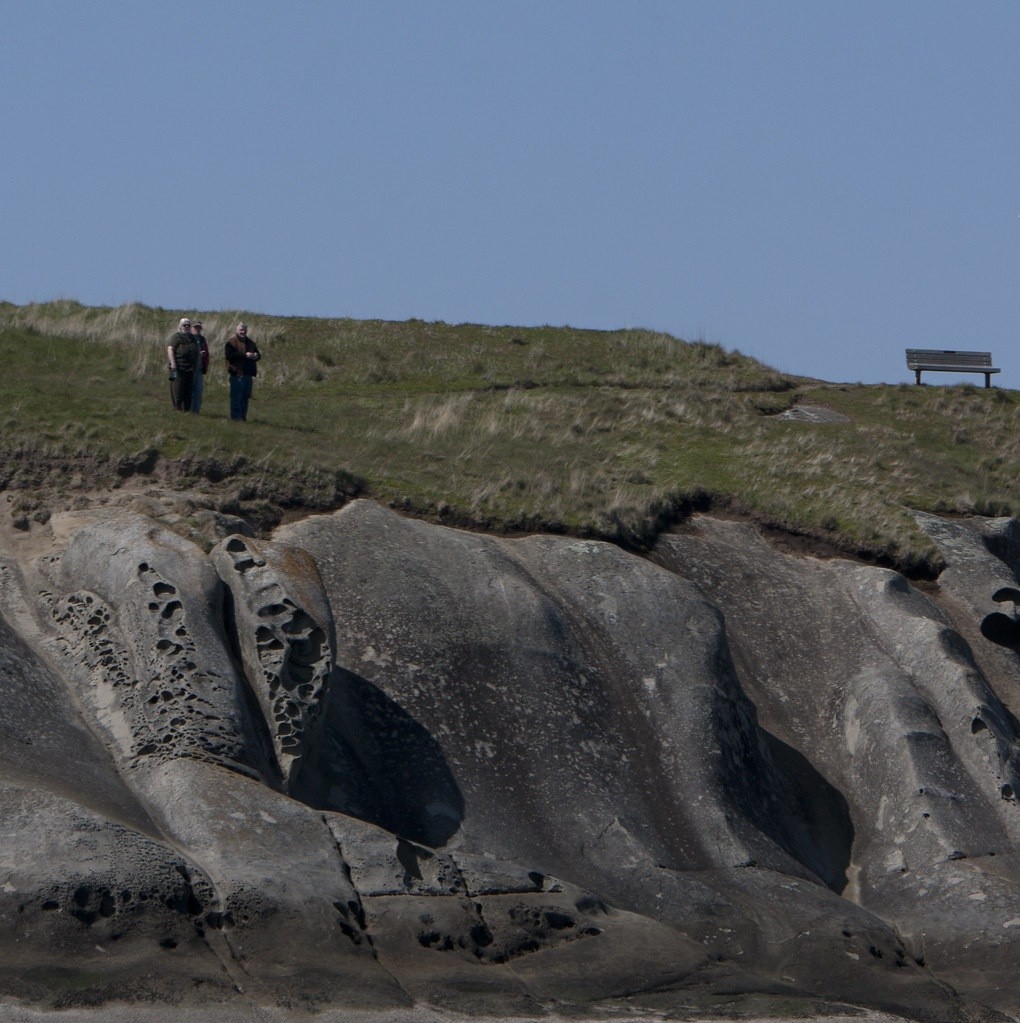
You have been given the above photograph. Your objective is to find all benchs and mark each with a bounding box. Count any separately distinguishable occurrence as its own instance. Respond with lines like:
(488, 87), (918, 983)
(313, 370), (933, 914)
(905, 348), (1000, 389)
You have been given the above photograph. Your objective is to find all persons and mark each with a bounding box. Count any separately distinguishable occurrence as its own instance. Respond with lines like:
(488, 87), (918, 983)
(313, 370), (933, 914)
(191, 319), (209, 415)
(167, 318), (198, 412)
(225, 322), (261, 422)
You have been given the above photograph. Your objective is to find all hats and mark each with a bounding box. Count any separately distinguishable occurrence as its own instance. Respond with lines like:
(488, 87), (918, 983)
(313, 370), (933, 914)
(192, 320), (203, 329)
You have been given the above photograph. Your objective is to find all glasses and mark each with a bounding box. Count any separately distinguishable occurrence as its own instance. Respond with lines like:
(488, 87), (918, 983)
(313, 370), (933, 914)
(181, 324), (190, 327)
